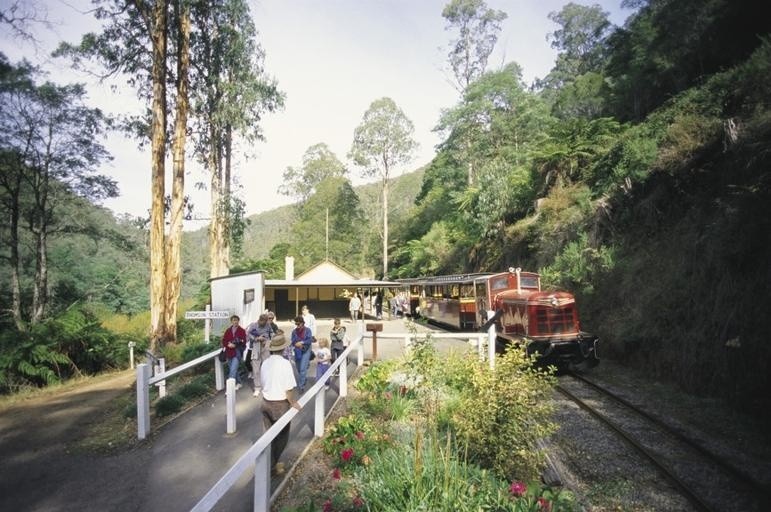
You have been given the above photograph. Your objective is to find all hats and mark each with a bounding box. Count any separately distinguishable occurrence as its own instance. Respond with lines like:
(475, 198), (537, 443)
(268, 334), (292, 352)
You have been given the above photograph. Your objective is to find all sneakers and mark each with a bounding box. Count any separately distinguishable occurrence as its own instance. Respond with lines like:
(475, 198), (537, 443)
(236, 382), (242, 390)
(253, 387), (260, 396)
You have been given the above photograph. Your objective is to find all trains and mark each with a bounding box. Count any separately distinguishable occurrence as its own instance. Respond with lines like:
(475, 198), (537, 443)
(383, 268), (601, 376)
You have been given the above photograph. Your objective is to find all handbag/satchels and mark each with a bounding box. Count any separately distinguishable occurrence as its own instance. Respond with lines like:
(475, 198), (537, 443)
(294, 348), (302, 360)
(219, 350), (227, 362)
(334, 340), (344, 350)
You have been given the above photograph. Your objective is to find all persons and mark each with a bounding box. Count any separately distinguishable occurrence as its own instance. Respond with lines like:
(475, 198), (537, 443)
(329, 319), (343, 376)
(259, 335), (303, 473)
(341, 327), (351, 351)
(315, 337), (332, 391)
(348, 287), (410, 321)
(221, 305), (316, 397)
